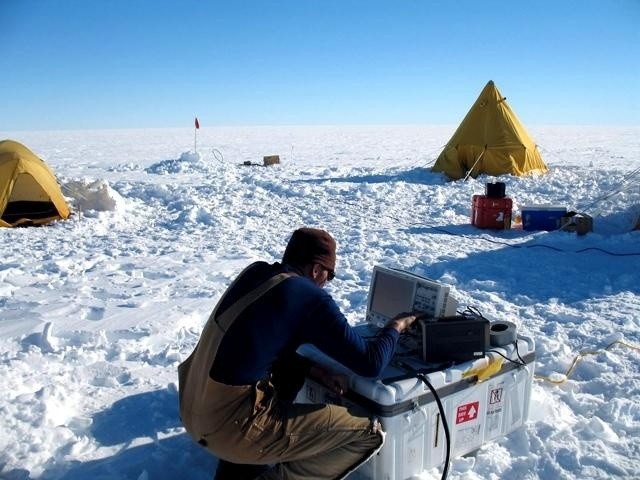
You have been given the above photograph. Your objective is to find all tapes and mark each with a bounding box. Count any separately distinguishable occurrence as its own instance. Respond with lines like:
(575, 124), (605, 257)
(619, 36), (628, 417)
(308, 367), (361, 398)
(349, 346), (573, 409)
(490, 321), (517, 346)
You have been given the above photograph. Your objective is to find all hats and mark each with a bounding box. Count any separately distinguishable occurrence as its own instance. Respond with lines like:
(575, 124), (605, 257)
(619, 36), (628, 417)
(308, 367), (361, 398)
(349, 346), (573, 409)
(283, 228), (338, 273)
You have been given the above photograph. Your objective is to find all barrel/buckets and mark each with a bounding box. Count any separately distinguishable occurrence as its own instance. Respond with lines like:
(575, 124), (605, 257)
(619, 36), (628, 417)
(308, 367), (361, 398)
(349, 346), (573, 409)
(485, 182), (506, 198)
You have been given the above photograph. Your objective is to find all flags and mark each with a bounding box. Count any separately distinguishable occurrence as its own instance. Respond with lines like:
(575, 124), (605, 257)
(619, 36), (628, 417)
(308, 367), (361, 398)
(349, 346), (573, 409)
(195, 118), (199, 128)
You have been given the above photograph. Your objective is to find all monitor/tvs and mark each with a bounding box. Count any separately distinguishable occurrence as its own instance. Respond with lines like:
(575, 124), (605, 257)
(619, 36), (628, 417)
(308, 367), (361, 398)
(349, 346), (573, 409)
(366, 264), (459, 335)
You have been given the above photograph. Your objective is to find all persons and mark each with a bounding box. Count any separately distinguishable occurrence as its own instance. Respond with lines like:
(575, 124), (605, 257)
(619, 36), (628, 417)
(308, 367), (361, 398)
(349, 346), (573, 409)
(178, 228), (427, 480)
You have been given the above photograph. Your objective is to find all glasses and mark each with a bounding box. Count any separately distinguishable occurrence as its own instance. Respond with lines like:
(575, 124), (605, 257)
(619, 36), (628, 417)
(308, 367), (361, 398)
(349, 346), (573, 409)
(328, 270), (336, 281)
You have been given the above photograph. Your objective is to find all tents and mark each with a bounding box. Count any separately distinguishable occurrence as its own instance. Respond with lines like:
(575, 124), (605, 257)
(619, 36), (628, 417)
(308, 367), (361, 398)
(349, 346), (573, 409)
(431, 80), (550, 180)
(1, 139), (71, 228)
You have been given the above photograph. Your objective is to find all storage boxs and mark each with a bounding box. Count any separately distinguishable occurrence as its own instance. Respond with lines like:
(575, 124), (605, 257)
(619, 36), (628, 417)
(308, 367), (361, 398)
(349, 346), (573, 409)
(294, 325), (535, 479)
(523, 207), (566, 231)
(470, 196), (512, 230)
(264, 156), (279, 166)
